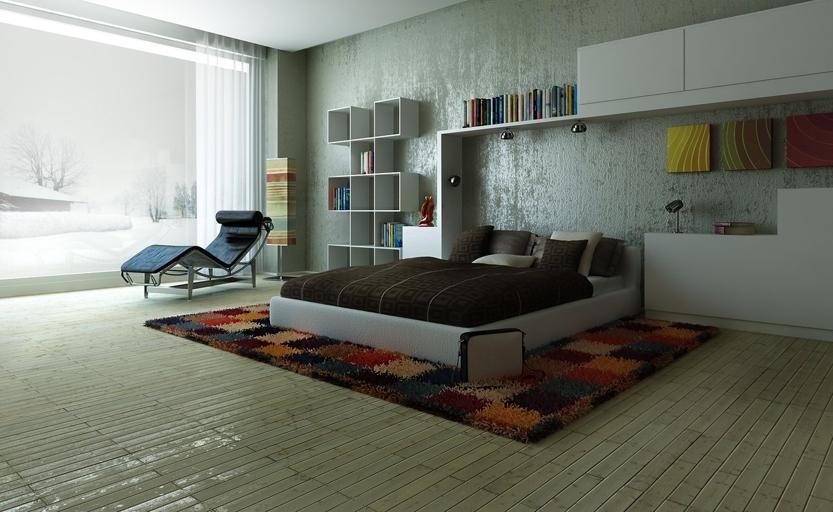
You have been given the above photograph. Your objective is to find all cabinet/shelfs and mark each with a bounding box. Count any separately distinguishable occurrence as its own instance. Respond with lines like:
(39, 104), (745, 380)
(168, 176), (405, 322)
(577, 0), (833, 119)
(327, 97), (420, 272)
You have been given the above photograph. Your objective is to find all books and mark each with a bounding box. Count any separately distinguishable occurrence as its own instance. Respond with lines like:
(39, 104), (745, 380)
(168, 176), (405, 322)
(358, 150), (374, 174)
(329, 186), (350, 210)
(463, 84), (576, 130)
(379, 220), (406, 247)
(712, 221), (756, 236)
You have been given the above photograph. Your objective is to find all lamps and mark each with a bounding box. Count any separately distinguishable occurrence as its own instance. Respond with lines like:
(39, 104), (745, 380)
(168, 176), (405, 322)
(501, 130), (514, 139)
(448, 175), (460, 187)
(571, 123), (587, 133)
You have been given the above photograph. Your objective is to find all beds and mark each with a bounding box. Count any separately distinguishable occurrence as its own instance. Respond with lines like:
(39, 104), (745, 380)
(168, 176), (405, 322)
(270, 229), (642, 369)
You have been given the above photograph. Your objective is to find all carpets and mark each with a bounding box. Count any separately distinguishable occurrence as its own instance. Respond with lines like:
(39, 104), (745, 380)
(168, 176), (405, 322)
(142, 302), (720, 445)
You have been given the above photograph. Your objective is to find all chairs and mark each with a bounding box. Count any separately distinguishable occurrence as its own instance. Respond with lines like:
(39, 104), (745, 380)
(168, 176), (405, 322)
(120, 210), (274, 300)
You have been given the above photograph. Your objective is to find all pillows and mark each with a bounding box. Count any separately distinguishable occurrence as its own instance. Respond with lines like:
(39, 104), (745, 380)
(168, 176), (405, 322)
(549, 228), (603, 277)
(448, 225), (494, 262)
(538, 238), (587, 274)
(488, 227), (532, 255)
(589, 236), (625, 277)
(471, 254), (537, 267)
